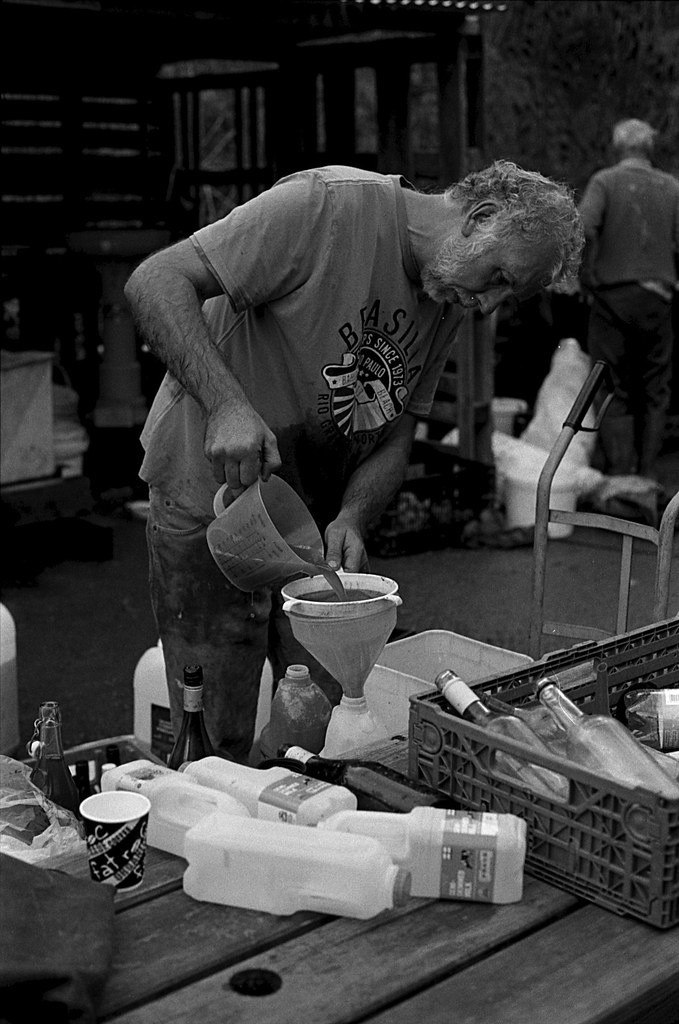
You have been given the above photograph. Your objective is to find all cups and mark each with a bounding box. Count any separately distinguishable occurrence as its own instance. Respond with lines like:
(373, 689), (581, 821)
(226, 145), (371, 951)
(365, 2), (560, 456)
(207, 473), (325, 593)
(79, 791), (151, 893)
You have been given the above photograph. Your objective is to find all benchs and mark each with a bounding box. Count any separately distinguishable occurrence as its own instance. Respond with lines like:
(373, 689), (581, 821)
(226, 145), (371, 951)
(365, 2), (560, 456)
(103, 727), (679, 1024)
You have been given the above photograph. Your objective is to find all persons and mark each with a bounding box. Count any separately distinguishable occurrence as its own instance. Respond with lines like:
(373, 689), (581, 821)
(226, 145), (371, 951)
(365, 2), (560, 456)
(121, 156), (583, 770)
(570, 118), (679, 524)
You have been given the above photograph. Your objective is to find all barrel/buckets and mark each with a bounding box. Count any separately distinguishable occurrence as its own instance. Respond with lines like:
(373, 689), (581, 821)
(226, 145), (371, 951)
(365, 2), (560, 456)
(505, 473), (578, 540)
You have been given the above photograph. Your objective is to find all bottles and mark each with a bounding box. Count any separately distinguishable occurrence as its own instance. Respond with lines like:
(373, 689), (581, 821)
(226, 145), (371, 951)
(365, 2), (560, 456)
(532, 677), (679, 797)
(168, 664), (214, 771)
(32, 702), (83, 821)
(433, 669), (568, 802)
(473, 689), (568, 757)
(317, 806), (526, 903)
(625, 689), (679, 749)
(183, 810), (411, 921)
(1, 602), (20, 754)
(177, 756), (357, 826)
(277, 743), (463, 812)
(101, 759), (250, 858)
(639, 741), (679, 780)
(258, 665), (333, 755)
(324, 694), (379, 757)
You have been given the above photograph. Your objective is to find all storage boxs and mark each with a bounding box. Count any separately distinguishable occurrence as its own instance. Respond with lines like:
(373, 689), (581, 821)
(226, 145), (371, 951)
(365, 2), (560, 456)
(313, 439), (499, 557)
(364, 629), (535, 732)
(408, 614), (679, 931)
(0, 350), (58, 487)
